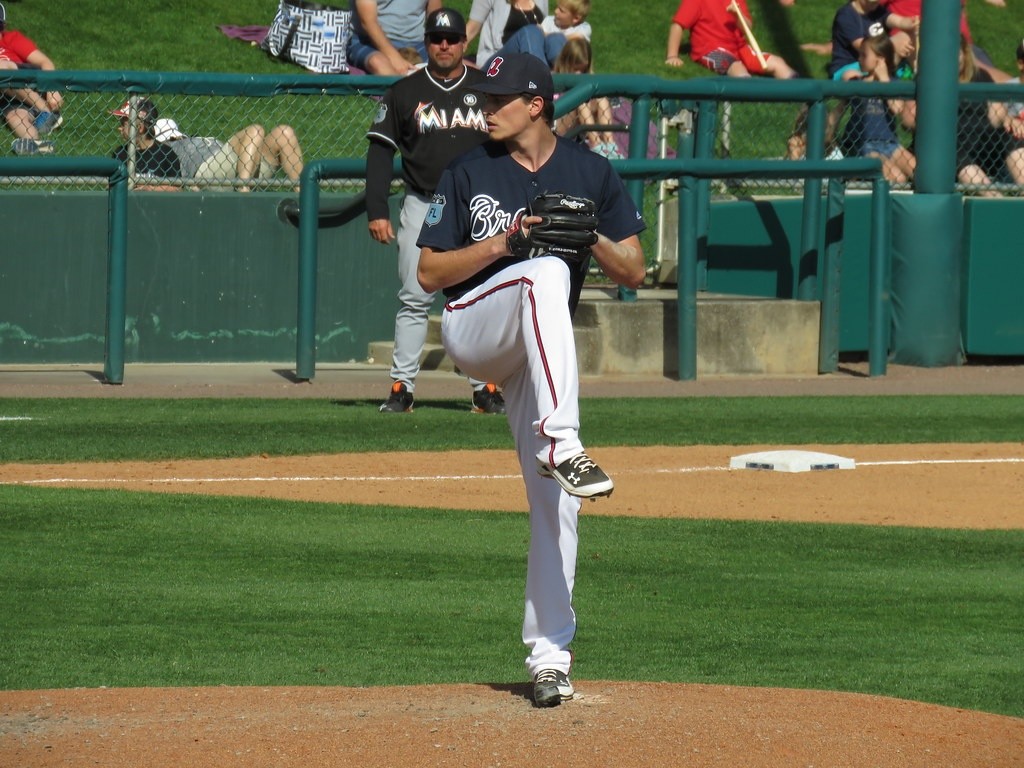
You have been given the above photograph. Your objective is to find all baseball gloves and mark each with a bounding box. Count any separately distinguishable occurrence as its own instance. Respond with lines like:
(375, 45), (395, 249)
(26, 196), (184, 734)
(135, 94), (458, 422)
(509, 193), (600, 275)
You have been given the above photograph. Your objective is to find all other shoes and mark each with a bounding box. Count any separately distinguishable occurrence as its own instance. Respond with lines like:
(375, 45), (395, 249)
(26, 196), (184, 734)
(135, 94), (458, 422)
(590, 141), (625, 160)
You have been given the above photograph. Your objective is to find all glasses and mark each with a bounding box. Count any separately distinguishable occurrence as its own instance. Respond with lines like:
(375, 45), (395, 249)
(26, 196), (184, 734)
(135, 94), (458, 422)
(429, 34), (460, 44)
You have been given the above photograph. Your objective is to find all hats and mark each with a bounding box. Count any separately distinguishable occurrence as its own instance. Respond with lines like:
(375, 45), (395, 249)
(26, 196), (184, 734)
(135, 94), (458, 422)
(107, 97), (158, 126)
(423, 8), (466, 37)
(462, 51), (555, 102)
(0, 3), (6, 24)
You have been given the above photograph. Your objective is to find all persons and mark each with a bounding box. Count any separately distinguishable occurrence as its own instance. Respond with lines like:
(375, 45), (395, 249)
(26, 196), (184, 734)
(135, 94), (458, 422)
(366, 8), (504, 413)
(346, 0), (478, 76)
(780, 0), (1024, 198)
(665, 0), (798, 78)
(154, 119), (303, 193)
(0, 18), (64, 156)
(109, 97), (183, 191)
(418, 52), (647, 705)
(462, 0), (628, 161)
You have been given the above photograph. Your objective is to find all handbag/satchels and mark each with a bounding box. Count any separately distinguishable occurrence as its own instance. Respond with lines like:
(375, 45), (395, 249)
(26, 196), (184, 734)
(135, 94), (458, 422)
(260, 0), (354, 74)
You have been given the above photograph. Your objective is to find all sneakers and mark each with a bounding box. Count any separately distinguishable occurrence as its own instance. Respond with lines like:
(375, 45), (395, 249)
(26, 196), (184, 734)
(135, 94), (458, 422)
(10, 137), (56, 155)
(379, 382), (414, 414)
(33, 111), (63, 137)
(470, 382), (508, 416)
(532, 668), (574, 708)
(537, 447), (615, 498)
